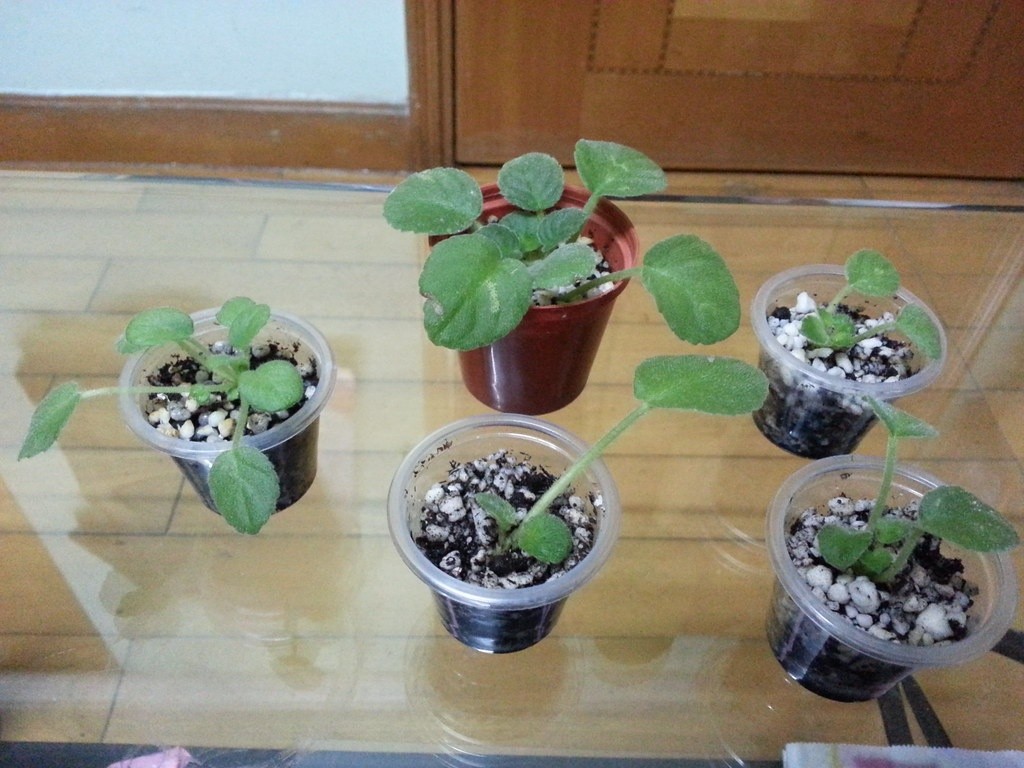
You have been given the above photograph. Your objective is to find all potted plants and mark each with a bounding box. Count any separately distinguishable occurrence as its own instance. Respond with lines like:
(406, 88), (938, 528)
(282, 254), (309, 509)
(765, 396), (1021, 703)
(757, 248), (950, 460)
(15, 295), (335, 536)
(383, 136), (740, 416)
(384, 350), (770, 649)
